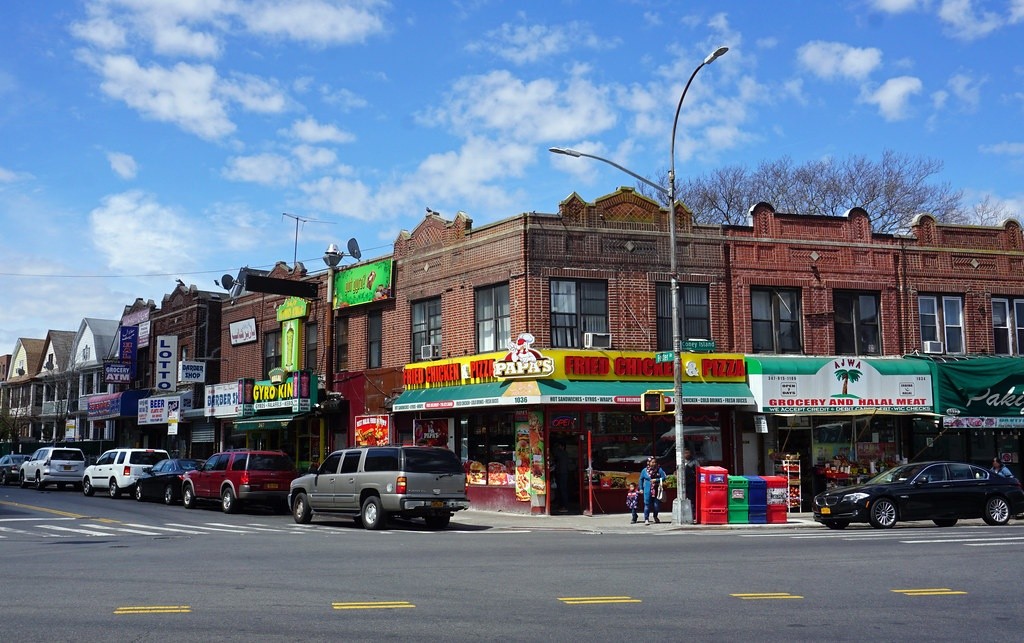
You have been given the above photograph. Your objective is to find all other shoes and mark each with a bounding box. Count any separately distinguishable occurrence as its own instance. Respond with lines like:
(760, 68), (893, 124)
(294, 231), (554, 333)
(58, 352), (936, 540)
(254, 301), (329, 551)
(654, 517), (659, 523)
(644, 519), (650, 525)
(630, 521), (636, 524)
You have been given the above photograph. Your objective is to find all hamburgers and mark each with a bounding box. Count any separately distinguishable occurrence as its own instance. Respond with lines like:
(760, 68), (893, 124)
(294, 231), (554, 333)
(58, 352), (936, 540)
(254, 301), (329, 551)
(469, 463), (483, 483)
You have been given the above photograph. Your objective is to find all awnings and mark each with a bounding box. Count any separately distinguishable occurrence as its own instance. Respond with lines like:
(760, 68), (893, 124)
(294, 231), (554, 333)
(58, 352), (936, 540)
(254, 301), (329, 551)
(232, 413), (304, 431)
(87, 389), (193, 425)
(744, 356), (935, 413)
(393, 380), (756, 412)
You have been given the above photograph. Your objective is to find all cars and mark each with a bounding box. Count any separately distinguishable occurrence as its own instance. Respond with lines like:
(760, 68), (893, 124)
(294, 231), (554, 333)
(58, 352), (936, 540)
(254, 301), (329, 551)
(134, 458), (207, 506)
(0, 454), (32, 486)
(811, 460), (1024, 530)
(601, 446), (625, 462)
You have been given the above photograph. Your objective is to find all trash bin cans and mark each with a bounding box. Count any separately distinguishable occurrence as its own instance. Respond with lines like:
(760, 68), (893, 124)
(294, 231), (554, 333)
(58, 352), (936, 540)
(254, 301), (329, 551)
(729, 476), (748, 523)
(695, 466), (728, 524)
(759, 476), (787, 523)
(744, 476), (767, 522)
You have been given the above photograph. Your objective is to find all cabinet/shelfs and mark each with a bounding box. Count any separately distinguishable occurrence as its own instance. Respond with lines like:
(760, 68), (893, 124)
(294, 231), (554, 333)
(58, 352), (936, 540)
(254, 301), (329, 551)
(774, 460), (803, 513)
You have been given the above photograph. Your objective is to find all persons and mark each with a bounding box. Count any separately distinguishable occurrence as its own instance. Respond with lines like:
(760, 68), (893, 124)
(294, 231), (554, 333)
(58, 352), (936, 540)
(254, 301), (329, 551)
(989, 457), (1014, 478)
(638, 456), (667, 525)
(685, 448), (700, 521)
(626, 482), (642, 524)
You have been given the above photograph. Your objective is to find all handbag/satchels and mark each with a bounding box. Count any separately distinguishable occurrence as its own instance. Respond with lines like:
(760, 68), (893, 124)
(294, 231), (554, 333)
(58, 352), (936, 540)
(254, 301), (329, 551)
(657, 478), (666, 503)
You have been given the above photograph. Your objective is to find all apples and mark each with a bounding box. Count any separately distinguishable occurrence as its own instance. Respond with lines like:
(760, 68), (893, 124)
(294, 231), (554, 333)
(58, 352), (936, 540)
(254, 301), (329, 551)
(787, 487), (800, 506)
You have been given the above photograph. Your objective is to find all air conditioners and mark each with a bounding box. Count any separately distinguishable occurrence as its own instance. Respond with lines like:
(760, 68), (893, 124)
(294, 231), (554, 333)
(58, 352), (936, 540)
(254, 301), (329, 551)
(924, 340), (943, 355)
(422, 345), (441, 361)
(689, 338), (709, 353)
(584, 332), (612, 349)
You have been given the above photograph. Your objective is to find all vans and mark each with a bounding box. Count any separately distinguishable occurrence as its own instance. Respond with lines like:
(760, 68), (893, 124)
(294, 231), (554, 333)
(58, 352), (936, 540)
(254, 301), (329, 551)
(618, 422), (722, 467)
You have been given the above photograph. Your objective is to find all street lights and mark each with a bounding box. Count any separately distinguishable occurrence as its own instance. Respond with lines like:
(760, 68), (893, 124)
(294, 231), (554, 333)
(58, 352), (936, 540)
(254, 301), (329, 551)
(547, 45), (730, 525)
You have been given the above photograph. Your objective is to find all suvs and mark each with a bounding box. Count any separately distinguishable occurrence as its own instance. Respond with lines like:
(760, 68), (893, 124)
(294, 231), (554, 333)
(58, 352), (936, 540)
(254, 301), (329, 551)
(181, 448), (301, 515)
(18, 446), (89, 492)
(287, 443), (471, 531)
(82, 449), (171, 499)
